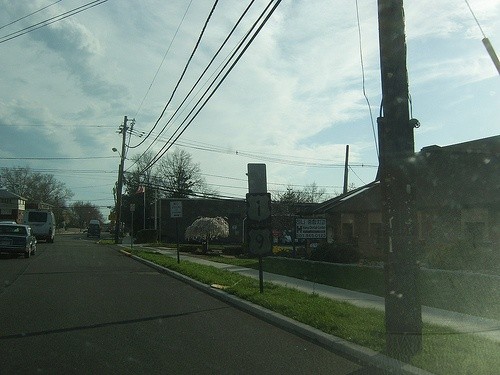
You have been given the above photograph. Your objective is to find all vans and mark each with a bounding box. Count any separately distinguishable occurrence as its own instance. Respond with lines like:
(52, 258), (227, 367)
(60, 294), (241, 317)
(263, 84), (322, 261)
(25, 207), (57, 242)
(86, 222), (101, 239)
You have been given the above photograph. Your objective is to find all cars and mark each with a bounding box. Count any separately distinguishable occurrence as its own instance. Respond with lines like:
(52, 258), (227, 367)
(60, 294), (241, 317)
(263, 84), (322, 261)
(0, 223), (38, 260)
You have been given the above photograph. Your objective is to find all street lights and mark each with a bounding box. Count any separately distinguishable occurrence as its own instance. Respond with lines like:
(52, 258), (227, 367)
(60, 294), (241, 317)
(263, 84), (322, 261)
(109, 148), (122, 242)
